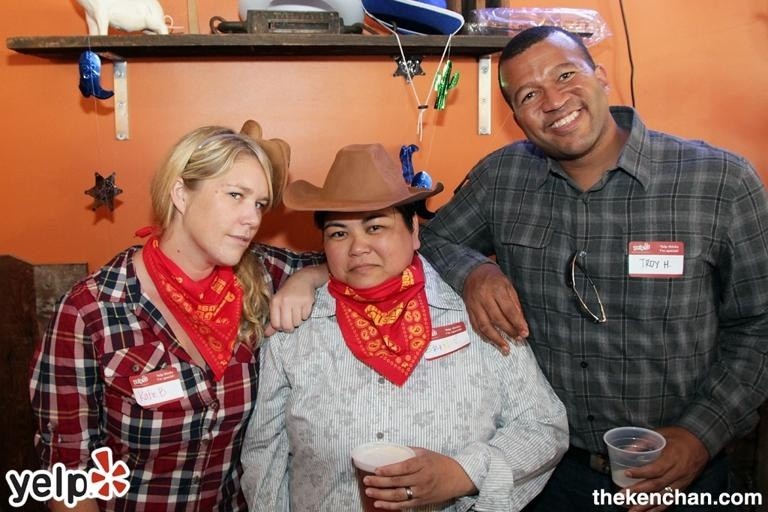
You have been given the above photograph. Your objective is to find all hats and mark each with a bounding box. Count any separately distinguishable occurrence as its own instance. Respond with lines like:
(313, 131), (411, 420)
(283, 145), (443, 212)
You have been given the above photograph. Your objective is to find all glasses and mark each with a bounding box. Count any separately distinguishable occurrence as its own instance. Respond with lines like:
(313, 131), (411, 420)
(567, 250), (607, 323)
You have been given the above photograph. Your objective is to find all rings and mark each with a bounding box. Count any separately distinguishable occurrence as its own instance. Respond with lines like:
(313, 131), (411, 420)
(406, 487), (413, 499)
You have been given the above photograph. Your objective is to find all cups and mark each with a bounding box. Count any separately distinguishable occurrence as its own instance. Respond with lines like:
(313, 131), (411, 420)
(351, 442), (419, 508)
(603, 427), (667, 488)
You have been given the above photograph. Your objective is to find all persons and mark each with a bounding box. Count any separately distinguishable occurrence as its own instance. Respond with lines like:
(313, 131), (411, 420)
(411, 26), (768, 508)
(240, 142), (571, 511)
(27, 127), (330, 511)
